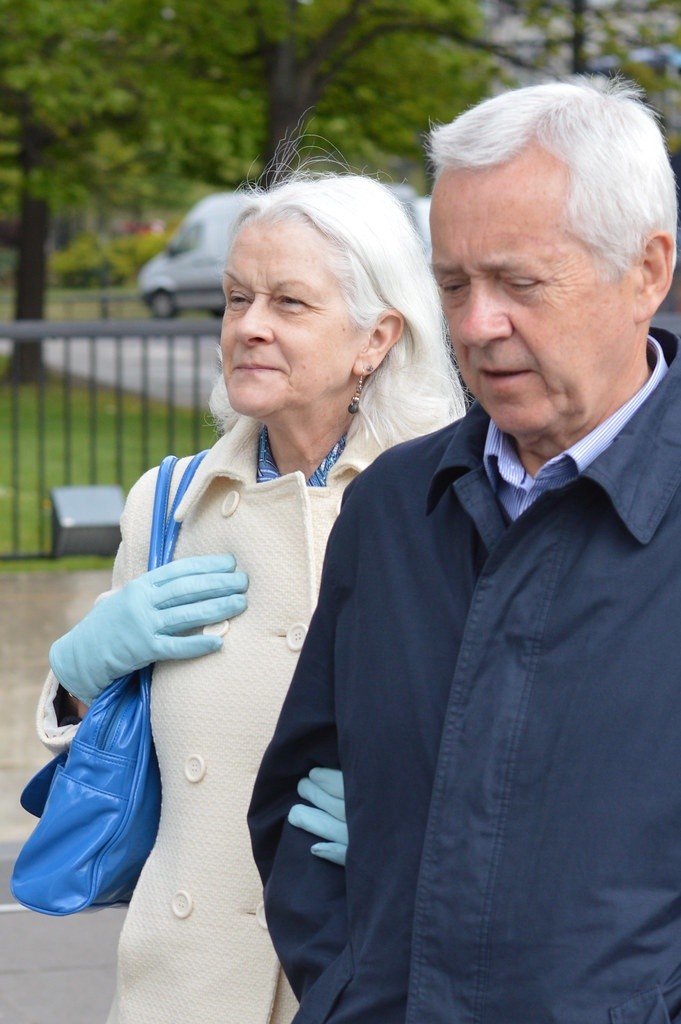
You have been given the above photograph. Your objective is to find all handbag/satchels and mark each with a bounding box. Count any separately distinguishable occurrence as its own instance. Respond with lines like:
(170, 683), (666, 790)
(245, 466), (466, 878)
(11, 446), (210, 915)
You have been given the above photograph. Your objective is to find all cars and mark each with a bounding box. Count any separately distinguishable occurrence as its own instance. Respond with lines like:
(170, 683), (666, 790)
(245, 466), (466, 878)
(140, 187), (274, 320)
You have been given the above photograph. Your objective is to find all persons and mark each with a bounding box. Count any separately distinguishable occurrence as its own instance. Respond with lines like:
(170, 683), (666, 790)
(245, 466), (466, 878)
(247, 75), (681, 1024)
(36, 176), (466, 1024)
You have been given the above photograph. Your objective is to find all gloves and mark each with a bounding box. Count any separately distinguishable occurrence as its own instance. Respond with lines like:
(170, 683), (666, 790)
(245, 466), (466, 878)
(288, 768), (350, 865)
(48, 554), (250, 708)
